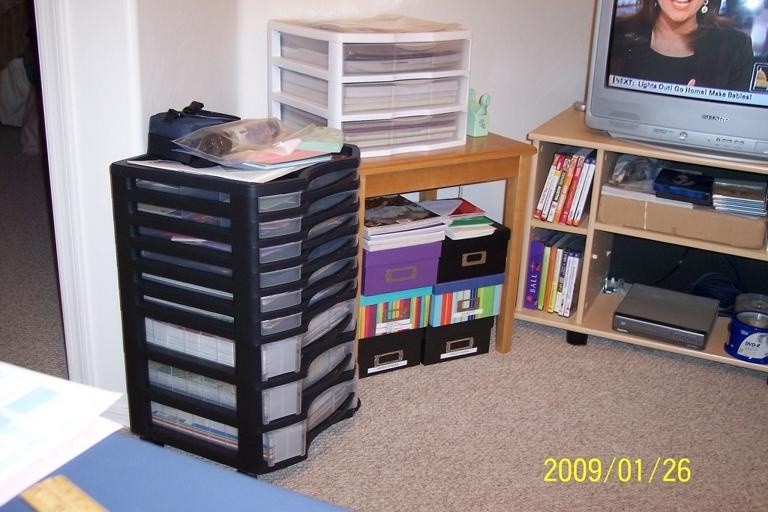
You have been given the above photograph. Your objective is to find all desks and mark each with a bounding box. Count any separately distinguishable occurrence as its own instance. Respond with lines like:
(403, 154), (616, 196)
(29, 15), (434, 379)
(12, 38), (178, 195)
(356, 129), (538, 353)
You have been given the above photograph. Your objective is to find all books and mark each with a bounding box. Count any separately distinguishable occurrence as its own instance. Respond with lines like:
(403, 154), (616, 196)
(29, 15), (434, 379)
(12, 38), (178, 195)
(651, 167), (768, 220)
(275, 33), (460, 150)
(358, 195), (498, 253)
(522, 144), (597, 317)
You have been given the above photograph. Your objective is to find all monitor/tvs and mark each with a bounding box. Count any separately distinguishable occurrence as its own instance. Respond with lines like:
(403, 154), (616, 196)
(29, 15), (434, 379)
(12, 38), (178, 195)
(585, 0), (768, 164)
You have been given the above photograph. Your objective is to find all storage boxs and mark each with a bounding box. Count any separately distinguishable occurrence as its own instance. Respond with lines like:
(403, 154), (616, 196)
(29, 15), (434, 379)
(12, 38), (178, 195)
(110, 143), (361, 475)
(357, 198), (511, 378)
(267, 16), (472, 159)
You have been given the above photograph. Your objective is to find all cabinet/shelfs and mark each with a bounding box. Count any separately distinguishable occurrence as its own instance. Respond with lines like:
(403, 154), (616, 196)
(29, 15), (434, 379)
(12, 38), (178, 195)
(513, 101), (768, 386)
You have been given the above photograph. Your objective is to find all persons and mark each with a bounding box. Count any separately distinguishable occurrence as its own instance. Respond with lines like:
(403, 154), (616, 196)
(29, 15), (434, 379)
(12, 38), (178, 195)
(607, 0), (756, 92)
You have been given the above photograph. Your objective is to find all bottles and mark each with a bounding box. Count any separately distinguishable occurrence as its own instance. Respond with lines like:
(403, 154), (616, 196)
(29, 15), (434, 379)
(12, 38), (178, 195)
(202, 118), (281, 157)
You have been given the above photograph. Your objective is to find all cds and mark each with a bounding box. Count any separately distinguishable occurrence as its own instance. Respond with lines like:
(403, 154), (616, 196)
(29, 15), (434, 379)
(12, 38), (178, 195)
(734, 292), (768, 314)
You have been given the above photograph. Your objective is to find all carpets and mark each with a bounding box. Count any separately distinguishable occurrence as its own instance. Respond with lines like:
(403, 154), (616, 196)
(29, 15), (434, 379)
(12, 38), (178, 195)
(118, 319), (768, 512)
